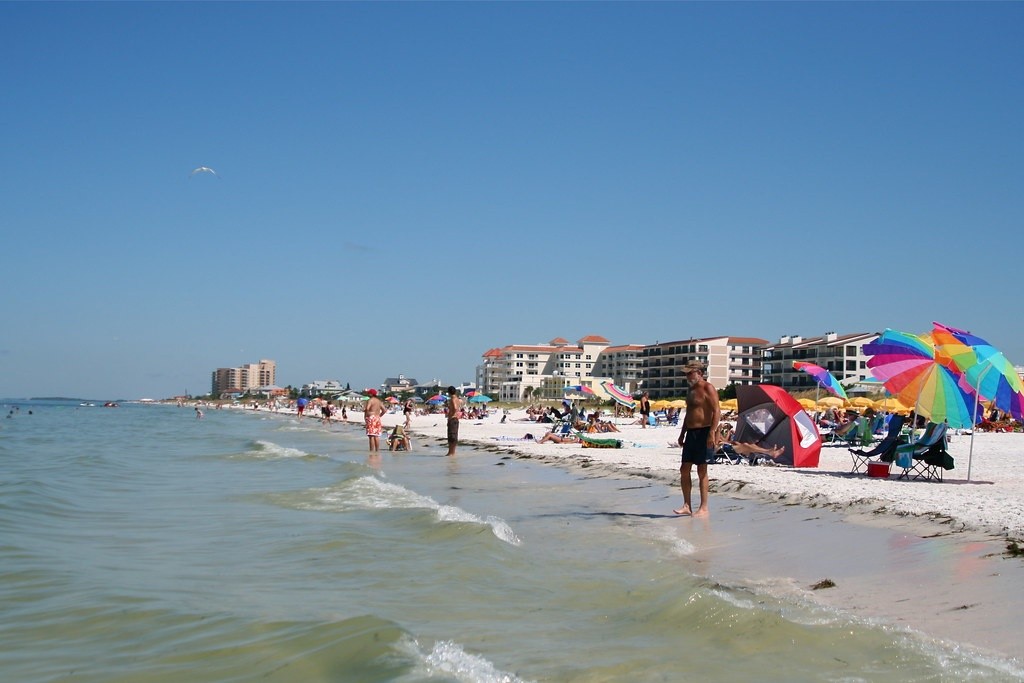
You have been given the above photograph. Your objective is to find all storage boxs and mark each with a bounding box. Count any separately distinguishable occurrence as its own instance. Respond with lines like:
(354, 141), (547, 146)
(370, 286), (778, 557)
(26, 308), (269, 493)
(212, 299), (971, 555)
(868, 461), (890, 477)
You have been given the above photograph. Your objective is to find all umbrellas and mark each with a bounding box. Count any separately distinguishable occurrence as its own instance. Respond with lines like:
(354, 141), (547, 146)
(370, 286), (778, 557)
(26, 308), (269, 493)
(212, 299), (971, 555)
(733, 385), (821, 468)
(561, 385), (598, 409)
(718, 398), (738, 410)
(792, 360), (850, 423)
(863, 328), (984, 428)
(268, 389), (492, 413)
(633, 400), (687, 411)
(929, 322), (1024, 483)
(600, 381), (637, 424)
(795, 395), (915, 415)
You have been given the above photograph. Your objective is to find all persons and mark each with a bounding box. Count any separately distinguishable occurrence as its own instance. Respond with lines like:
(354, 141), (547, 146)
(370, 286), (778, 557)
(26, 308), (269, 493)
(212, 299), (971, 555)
(716, 404), (927, 442)
(639, 390), (681, 429)
(987, 410), (1011, 422)
(364, 389), (386, 451)
(386, 425), (412, 452)
(673, 360), (720, 518)
(714, 423), (784, 460)
(402, 399), (412, 429)
(378, 401), (488, 419)
(444, 386), (459, 457)
(177, 395), (367, 426)
(500, 401), (636, 444)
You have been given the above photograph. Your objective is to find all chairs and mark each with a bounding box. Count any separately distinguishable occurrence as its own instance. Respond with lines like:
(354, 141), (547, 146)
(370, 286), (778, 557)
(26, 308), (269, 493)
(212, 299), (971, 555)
(711, 407), (954, 483)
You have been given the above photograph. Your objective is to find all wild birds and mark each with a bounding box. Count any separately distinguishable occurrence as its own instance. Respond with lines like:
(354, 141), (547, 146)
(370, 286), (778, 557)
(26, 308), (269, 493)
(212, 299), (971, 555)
(193, 167), (217, 175)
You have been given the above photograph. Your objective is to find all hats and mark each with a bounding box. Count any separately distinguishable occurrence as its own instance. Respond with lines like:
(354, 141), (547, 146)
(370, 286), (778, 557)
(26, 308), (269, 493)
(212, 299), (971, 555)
(680, 359), (704, 373)
(367, 389), (376, 396)
